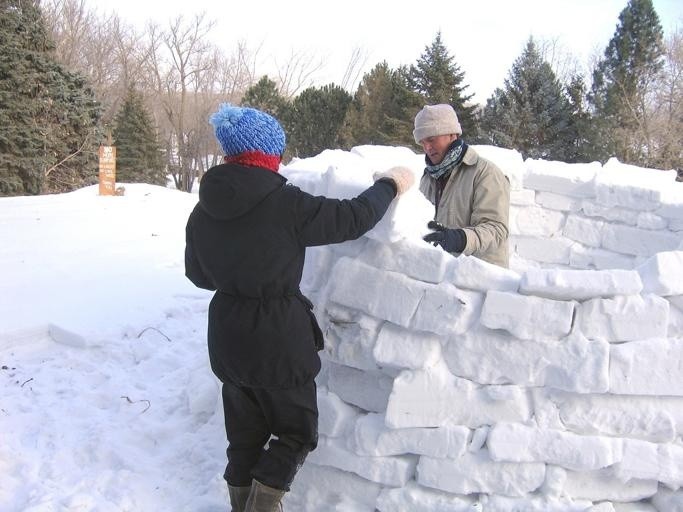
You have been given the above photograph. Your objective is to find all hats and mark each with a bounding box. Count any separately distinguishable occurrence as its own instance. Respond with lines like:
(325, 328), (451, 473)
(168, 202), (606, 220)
(413, 104), (463, 147)
(208, 101), (287, 158)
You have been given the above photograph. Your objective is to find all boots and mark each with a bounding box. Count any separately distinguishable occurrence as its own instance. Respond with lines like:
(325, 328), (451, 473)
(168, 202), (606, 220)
(227, 479), (291, 511)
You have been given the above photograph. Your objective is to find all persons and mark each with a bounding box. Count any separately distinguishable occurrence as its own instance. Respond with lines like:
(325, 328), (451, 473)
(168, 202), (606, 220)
(184, 104), (416, 512)
(413, 103), (512, 268)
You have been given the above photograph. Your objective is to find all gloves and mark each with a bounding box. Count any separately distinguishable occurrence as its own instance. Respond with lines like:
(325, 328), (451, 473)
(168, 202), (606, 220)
(424, 221), (466, 254)
(373, 165), (415, 198)
